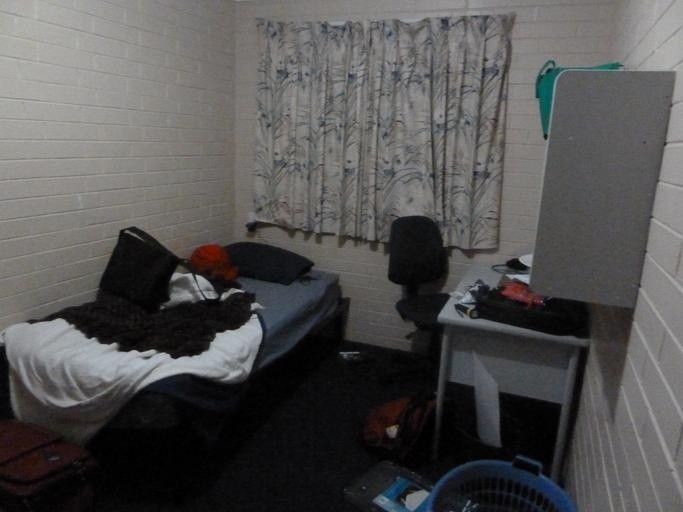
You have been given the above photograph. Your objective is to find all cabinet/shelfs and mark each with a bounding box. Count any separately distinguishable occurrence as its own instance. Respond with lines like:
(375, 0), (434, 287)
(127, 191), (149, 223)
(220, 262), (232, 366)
(529, 69), (676, 309)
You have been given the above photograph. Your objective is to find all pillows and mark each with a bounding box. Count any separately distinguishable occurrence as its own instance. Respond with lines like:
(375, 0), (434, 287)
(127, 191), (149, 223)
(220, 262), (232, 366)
(224, 242), (312, 285)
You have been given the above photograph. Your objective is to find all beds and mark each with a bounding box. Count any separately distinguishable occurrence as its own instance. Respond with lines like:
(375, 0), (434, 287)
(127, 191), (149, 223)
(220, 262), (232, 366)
(2, 268), (350, 447)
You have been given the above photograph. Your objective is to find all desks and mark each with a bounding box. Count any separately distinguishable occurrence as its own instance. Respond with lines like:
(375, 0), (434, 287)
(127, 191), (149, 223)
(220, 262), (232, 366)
(433, 265), (590, 484)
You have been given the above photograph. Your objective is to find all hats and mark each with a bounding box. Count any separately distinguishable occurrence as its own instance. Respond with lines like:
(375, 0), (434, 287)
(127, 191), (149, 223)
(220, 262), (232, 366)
(191, 245), (238, 283)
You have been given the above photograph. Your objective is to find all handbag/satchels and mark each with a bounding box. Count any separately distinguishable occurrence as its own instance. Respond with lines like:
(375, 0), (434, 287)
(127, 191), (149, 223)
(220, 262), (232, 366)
(362, 390), (436, 451)
(100, 227), (180, 313)
(0, 419), (98, 512)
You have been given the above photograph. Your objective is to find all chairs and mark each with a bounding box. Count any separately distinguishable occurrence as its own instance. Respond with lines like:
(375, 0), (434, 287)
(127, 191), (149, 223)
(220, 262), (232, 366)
(379, 216), (451, 398)
